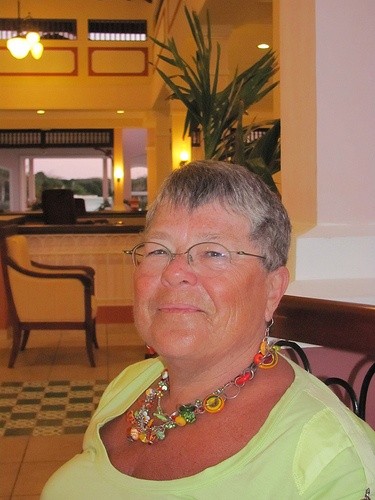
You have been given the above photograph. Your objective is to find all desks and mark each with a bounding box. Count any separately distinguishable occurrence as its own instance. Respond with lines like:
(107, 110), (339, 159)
(0, 210), (147, 323)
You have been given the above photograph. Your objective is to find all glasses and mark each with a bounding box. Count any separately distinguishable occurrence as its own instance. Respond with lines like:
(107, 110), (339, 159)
(124, 242), (264, 276)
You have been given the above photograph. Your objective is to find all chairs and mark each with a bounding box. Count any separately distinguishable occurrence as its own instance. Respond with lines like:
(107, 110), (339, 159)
(0, 233), (100, 370)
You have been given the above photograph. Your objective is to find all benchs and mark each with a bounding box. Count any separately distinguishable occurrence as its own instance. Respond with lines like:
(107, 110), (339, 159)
(268, 295), (375, 421)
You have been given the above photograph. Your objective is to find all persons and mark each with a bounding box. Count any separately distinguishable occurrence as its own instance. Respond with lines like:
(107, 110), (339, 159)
(40, 159), (375, 500)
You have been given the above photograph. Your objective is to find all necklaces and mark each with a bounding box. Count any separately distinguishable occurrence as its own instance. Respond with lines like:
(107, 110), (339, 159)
(127, 344), (280, 445)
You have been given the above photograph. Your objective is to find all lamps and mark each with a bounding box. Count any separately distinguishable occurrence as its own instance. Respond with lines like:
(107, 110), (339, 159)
(6, 0), (44, 62)
(114, 164), (123, 183)
(180, 153), (189, 165)
